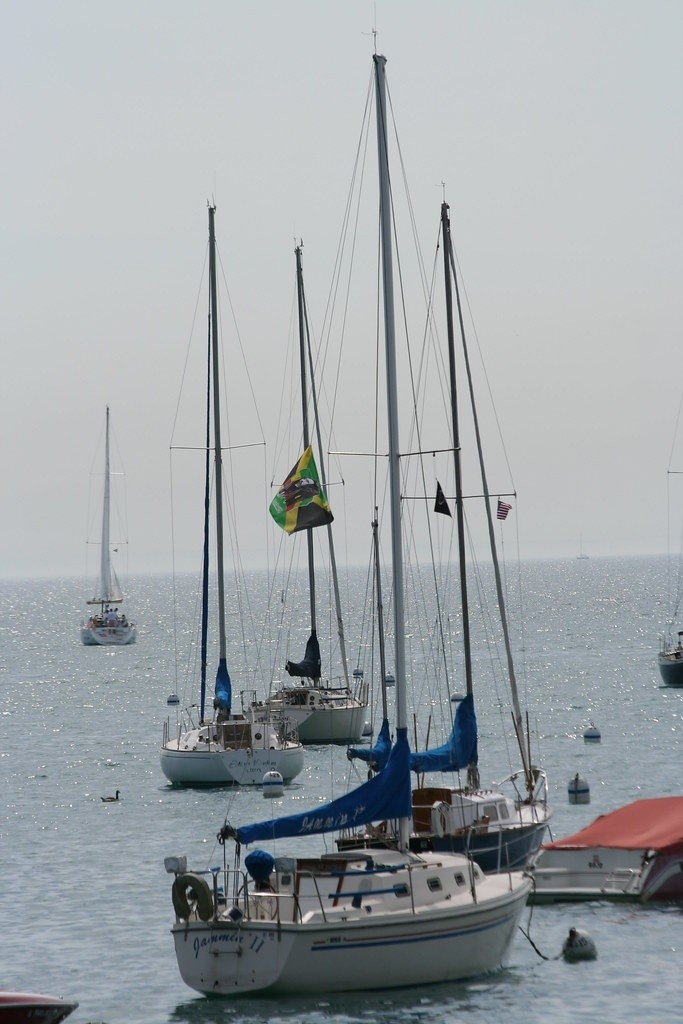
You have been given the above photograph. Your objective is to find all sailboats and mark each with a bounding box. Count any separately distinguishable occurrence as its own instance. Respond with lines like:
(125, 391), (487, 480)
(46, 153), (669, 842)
(79, 405), (137, 647)
(657, 391), (682, 690)
(160, 40), (557, 998)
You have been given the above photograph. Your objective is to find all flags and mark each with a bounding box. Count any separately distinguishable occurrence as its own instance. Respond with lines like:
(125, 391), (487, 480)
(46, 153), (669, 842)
(496, 500), (513, 520)
(433, 480), (452, 519)
(269, 444), (335, 536)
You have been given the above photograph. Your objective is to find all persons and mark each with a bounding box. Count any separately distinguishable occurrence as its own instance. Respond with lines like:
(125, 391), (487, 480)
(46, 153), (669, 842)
(92, 607), (127, 627)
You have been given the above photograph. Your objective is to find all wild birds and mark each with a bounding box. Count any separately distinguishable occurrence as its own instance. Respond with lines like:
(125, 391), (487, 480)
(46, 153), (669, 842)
(99, 789), (121, 802)
